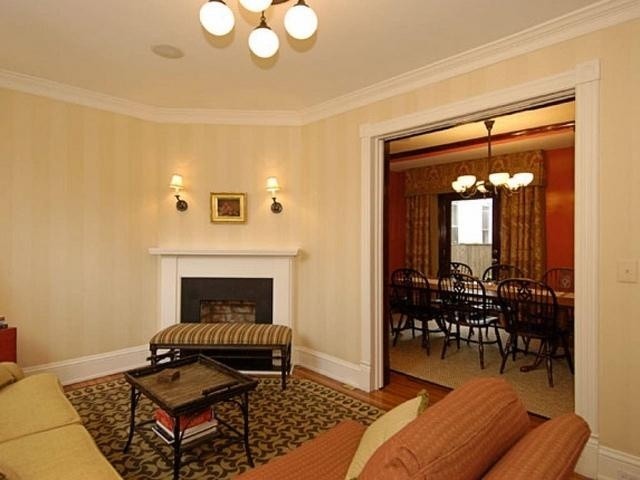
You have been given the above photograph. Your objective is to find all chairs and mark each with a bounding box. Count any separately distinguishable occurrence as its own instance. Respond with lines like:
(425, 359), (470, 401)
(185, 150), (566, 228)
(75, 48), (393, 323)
(389, 261), (573, 387)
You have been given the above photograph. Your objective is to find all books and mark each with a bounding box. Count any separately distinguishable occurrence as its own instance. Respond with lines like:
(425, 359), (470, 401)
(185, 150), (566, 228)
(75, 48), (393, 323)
(151, 418), (218, 447)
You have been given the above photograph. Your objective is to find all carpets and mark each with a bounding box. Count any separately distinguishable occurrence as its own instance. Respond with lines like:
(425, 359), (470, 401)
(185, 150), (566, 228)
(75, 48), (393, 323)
(388, 314), (574, 420)
(62, 365), (397, 480)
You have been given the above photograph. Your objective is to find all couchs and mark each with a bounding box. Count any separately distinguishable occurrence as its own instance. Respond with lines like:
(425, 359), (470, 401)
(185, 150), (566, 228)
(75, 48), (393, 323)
(228, 376), (591, 480)
(0, 361), (124, 480)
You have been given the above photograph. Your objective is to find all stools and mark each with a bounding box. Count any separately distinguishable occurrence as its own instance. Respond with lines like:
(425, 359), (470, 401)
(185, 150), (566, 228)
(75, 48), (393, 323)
(146, 324), (292, 392)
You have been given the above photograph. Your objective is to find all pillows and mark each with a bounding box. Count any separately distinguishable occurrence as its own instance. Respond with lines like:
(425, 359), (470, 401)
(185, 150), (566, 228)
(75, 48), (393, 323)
(344, 389), (428, 480)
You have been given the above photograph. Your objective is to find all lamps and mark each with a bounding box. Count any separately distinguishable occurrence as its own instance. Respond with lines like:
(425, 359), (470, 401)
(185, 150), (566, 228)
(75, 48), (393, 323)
(265, 177), (283, 213)
(200, 0), (320, 60)
(452, 120), (535, 199)
(169, 175), (189, 212)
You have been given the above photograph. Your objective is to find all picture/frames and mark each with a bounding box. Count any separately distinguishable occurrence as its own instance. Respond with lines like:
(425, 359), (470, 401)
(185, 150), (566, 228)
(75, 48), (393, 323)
(210, 192), (248, 224)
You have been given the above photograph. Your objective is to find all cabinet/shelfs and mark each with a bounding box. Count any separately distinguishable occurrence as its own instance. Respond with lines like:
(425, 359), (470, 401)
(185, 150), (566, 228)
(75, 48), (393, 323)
(0, 327), (16, 363)
